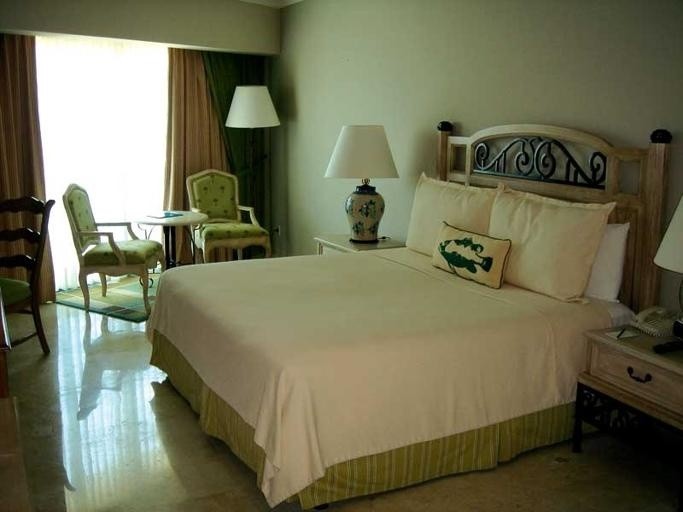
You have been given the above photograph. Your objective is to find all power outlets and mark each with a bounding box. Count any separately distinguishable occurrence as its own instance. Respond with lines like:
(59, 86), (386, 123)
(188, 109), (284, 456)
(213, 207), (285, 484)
(273, 223), (282, 236)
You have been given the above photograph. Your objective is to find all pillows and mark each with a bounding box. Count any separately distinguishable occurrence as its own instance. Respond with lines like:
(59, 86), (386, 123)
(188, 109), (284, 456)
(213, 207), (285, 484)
(404, 171), (632, 304)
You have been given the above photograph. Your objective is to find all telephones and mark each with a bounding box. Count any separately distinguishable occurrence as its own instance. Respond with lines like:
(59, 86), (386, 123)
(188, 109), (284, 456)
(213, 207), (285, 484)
(629, 305), (679, 337)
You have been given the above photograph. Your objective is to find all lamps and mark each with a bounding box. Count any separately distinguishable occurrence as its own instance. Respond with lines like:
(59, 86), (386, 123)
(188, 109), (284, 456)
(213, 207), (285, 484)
(321, 124), (400, 244)
(653, 192), (683, 339)
(224, 86), (281, 227)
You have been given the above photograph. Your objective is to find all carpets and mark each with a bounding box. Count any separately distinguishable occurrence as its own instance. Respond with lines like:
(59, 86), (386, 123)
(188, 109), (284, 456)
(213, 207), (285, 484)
(56, 271), (158, 323)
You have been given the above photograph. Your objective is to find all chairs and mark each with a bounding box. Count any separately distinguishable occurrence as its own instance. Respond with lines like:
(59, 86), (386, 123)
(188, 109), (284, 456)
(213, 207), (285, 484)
(185, 168), (271, 264)
(0, 194), (51, 356)
(61, 183), (167, 315)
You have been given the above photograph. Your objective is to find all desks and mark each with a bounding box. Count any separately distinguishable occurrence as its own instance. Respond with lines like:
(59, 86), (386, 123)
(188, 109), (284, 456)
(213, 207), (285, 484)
(146, 210), (209, 264)
(0, 291), (12, 398)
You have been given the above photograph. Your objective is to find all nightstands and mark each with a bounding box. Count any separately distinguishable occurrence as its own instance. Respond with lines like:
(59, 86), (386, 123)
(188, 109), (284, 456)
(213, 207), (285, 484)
(312, 232), (407, 254)
(569, 330), (683, 455)
